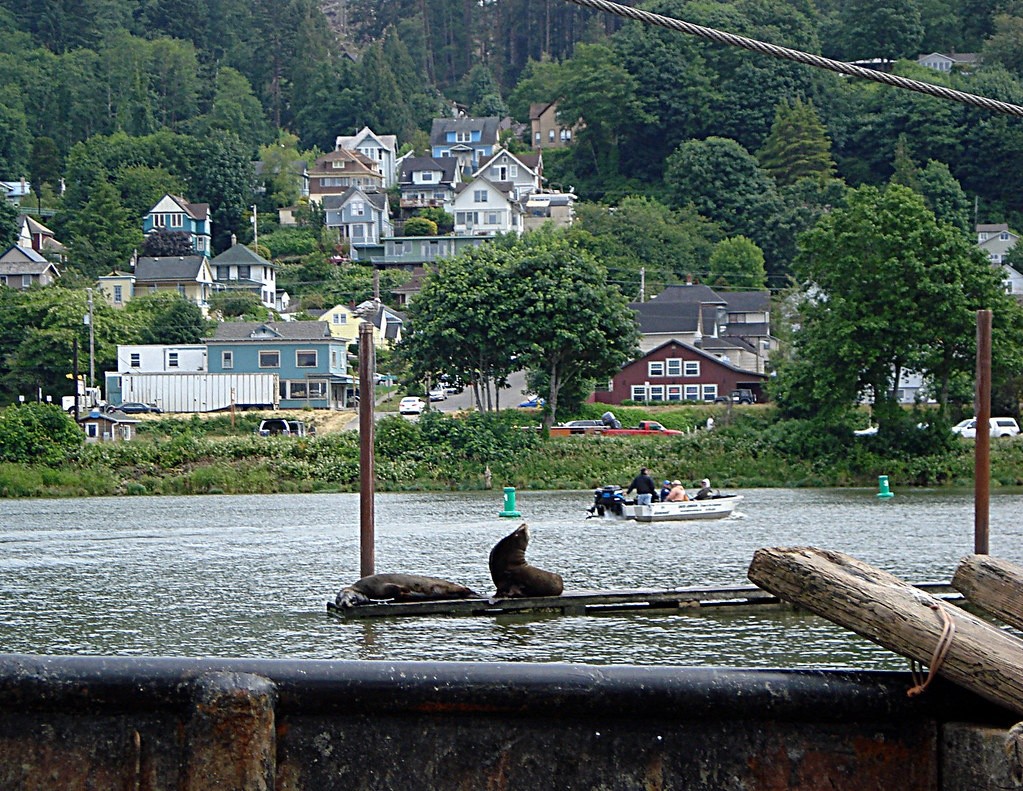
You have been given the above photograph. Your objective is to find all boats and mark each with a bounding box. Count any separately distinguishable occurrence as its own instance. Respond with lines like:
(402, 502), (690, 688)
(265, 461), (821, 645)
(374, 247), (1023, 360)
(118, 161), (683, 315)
(620, 494), (746, 523)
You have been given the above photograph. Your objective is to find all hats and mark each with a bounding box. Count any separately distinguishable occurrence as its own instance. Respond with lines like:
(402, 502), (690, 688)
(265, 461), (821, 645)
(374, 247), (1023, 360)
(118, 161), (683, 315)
(663, 480), (671, 485)
(671, 479), (681, 485)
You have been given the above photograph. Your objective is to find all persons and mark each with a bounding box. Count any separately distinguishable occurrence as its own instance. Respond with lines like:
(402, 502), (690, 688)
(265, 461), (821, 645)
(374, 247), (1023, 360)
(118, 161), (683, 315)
(622, 468), (659, 505)
(690, 478), (712, 501)
(661, 480), (685, 502)
(707, 416), (713, 429)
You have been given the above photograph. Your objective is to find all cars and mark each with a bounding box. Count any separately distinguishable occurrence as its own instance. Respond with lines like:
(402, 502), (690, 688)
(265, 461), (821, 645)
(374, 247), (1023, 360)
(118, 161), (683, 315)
(113, 402), (164, 415)
(372, 373), (385, 385)
(399, 396), (425, 414)
(517, 396), (546, 408)
(425, 384), (448, 401)
(437, 382), (459, 394)
(258, 417), (306, 440)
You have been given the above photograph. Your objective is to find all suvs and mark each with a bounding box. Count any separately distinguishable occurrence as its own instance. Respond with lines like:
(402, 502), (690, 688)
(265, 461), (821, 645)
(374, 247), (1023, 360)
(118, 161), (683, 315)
(715, 388), (757, 406)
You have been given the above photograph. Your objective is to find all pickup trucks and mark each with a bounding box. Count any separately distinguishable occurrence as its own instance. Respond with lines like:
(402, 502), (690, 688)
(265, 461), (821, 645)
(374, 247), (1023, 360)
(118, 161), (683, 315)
(602, 420), (685, 437)
(950, 416), (1020, 439)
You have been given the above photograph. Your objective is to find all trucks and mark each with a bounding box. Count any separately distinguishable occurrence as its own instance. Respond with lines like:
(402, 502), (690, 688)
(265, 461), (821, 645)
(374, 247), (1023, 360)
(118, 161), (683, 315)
(61, 396), (109, 416)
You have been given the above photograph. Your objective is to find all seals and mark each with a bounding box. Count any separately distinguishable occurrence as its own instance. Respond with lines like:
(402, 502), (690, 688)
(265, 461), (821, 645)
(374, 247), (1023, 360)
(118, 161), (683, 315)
(336, 574), (490, 607)
(489, 524), (563, 598)
